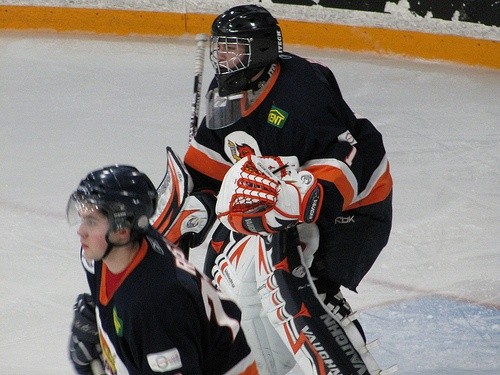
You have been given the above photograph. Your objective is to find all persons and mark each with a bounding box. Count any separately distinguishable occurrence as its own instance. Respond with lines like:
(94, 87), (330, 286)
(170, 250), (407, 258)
(68, 165), (265, 375)
(173, 5), (393, 374)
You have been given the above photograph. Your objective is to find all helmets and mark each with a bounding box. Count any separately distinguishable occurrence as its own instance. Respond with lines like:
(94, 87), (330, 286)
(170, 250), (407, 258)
(209, 4), (284, 97)
(71, 165), (159, 231)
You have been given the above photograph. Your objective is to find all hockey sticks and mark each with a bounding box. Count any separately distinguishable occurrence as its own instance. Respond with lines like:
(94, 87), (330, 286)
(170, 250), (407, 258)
(91, 359), (106, 375)
(177, 34), (208, 261)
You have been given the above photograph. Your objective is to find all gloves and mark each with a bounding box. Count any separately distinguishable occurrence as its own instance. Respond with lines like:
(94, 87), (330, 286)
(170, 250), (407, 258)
(70, 293), (103, 367)
